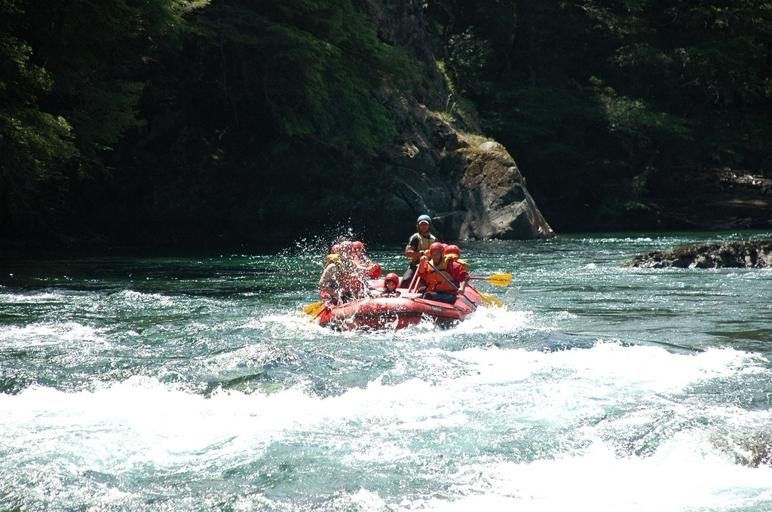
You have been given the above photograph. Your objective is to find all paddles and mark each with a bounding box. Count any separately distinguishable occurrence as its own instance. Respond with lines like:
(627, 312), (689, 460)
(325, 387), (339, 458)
(470, 274), (512, 286)
(304, 302), (323, 316)
(466, 284), (503, 308)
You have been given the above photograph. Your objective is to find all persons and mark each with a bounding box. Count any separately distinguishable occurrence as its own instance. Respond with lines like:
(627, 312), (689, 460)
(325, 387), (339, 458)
(319, 215), (470, 304)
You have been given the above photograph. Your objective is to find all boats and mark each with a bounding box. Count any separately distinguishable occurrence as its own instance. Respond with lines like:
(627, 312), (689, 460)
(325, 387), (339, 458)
(319, 278), (481, 334)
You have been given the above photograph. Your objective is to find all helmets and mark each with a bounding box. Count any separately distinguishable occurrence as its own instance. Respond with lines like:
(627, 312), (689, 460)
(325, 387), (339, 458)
(384, 273), (399, 290)
(331, 241), (363, 256)
(417, 215), (432, 227)
(430, 242), (461, 258)
